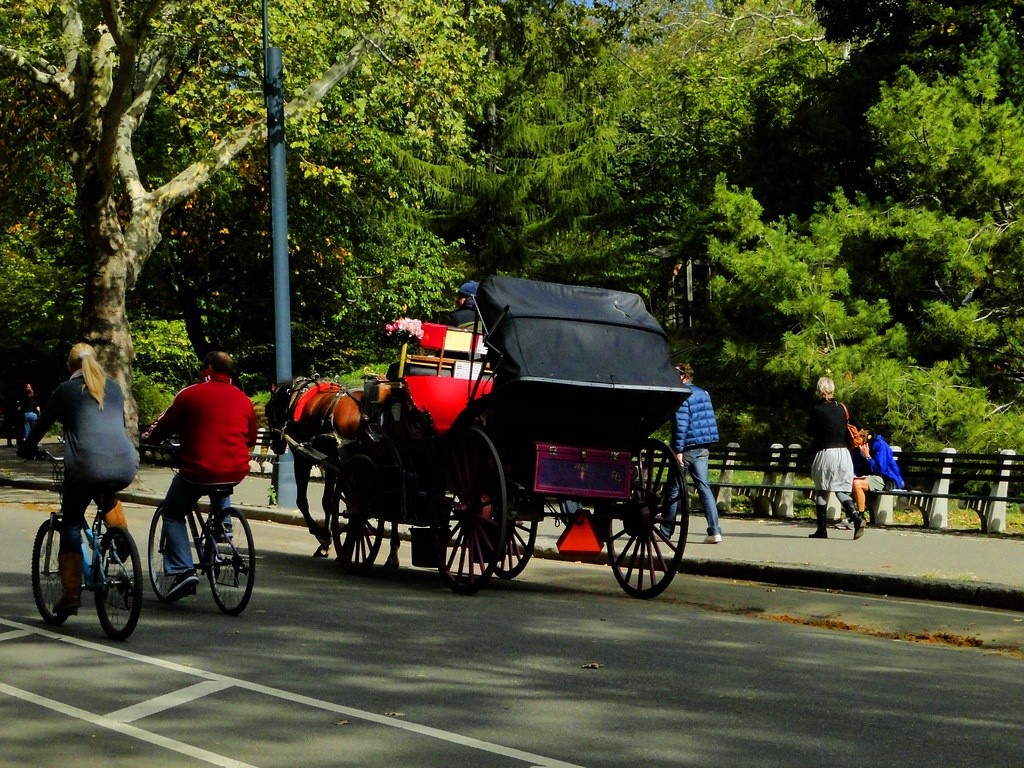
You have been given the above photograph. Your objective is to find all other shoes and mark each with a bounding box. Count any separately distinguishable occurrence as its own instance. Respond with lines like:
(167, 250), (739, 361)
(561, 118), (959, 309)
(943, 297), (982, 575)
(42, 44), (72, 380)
(834, 518), (855, 529)
(703, 534), (722, 543)
(649, 530), (669, 542)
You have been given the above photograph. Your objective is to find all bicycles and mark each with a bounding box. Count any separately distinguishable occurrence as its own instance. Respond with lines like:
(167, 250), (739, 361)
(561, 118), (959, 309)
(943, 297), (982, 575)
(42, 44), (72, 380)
(18, 448), (145, 642)
(144, 437), (256, 617)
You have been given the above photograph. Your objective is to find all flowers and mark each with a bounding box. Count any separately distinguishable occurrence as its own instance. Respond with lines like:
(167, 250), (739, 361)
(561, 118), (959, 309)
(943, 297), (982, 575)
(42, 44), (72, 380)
(386, 317), (423, 340)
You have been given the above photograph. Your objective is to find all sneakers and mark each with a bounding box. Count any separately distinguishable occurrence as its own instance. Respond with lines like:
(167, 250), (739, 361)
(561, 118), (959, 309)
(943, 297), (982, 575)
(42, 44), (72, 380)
(165, 569), (199, 601)
(215, 524), (235, 539)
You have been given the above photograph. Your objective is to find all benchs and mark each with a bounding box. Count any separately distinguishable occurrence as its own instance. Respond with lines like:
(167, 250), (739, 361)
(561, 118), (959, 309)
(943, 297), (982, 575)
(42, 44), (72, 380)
(136, 424), (270, 473)
(405, 375), (494, 438)
(639, 441), (1024, 533)
(385, 321), (488, 382)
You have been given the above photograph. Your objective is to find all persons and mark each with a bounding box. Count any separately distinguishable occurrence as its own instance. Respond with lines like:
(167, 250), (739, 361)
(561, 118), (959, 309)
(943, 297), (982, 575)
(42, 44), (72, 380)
(18, 342), (139, 614)
(139, 351), (256, 602)
(18, 383), (41, 446)
(653, 362), (721, 544)
(807, 374), (867, 541)
(387, 344), (425, 382)
(434, 279), (488, 358)
(835, 429), (906, 530)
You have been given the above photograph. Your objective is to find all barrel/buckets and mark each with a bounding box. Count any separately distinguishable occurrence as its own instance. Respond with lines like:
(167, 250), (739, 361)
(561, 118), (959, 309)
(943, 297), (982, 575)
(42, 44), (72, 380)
(409, 527), (450, 567)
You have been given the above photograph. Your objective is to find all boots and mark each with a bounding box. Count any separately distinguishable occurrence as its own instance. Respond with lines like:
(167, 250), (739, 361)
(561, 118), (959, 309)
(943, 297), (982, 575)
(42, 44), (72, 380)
(809, 504), (827, 538)
(100, 499), (132, 564)
(842, 500), (866, 540)
(54, 549), (83, 613)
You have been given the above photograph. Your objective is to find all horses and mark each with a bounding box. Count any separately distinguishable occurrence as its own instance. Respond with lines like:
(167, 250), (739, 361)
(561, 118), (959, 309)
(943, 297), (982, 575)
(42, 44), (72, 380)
(265, 380), (401, 570)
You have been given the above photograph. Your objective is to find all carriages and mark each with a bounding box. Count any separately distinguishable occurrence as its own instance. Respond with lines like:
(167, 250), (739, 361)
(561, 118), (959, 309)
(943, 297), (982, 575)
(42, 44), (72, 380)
(264, 273), (694, 600)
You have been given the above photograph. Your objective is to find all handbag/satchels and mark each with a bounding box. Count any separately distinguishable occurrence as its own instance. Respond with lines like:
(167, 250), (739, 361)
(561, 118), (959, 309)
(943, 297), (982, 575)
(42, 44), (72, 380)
(839, 402), (863, 450)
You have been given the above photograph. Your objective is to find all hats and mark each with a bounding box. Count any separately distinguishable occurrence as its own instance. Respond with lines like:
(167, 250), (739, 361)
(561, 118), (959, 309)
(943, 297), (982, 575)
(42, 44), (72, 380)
(458, 281), (478, 296)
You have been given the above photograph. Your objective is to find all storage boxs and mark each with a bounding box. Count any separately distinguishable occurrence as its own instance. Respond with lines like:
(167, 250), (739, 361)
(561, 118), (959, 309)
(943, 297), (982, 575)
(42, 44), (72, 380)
(524, 440), (631, 500)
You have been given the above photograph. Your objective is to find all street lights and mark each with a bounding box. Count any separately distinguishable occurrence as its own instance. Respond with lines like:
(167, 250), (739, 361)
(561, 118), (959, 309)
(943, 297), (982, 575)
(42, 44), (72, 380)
(216, 46), (297, 507)
